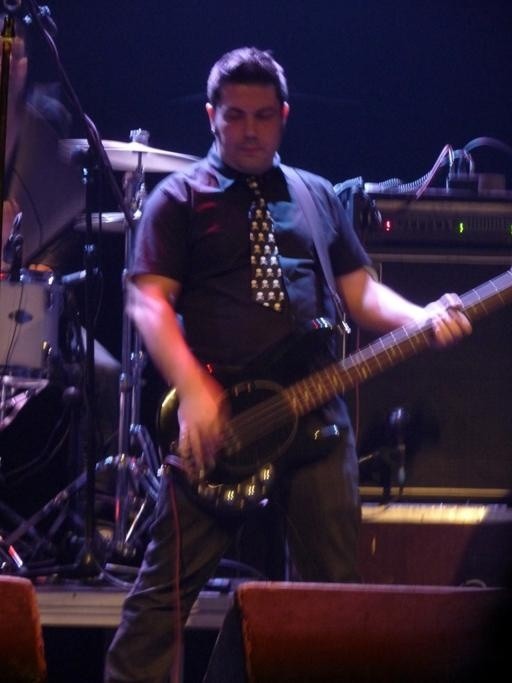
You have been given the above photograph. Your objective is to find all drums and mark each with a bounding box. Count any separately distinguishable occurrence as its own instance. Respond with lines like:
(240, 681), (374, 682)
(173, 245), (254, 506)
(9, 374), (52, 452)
(0, 268), (62, 369)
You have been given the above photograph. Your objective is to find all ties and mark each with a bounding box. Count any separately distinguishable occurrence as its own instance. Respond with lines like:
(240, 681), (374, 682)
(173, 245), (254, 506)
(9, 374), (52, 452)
(247, 176), (287, 314)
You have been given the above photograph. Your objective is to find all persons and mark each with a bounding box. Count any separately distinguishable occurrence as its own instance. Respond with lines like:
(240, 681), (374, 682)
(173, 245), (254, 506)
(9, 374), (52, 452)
(105, 47), (473, 683)
(0, 34), (85, 273)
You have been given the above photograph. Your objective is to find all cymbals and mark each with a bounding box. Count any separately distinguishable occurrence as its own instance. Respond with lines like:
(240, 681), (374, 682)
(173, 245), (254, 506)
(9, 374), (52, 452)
(57, 139), (202, 172)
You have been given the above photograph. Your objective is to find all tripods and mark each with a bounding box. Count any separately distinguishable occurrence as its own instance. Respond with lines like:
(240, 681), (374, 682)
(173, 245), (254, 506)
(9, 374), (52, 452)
(0, 146), (144, 591)
(103, 164), (264, 591)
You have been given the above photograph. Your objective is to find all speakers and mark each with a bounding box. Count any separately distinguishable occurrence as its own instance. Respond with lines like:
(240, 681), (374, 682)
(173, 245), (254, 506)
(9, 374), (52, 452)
(238, 581), (512, 683)
(349, 254), (511, 504)
(0, 574), (48, 683)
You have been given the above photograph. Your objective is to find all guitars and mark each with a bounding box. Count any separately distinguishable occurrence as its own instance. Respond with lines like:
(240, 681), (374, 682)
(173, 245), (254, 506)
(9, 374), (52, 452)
(160, 266), (511, 513)
